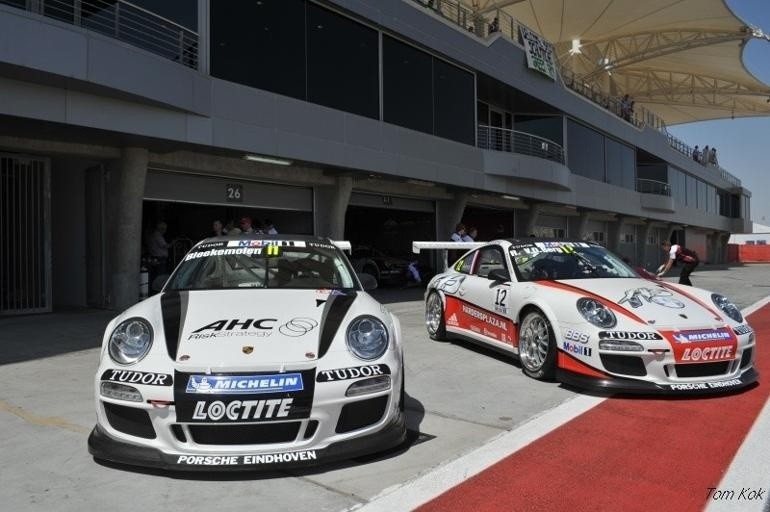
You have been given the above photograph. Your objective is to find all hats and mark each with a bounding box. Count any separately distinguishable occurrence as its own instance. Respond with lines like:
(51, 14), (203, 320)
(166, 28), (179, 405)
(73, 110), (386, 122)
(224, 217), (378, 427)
(239, 217), (252, 225)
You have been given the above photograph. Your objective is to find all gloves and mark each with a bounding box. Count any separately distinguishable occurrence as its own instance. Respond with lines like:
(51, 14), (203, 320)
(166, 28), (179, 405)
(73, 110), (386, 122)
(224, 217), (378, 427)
(656, 265), (669, 278)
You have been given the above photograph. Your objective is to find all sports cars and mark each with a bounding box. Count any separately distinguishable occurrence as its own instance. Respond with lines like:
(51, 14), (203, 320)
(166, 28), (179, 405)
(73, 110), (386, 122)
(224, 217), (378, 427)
(412, 240), (759, 397)
(87, 233), (407, 472)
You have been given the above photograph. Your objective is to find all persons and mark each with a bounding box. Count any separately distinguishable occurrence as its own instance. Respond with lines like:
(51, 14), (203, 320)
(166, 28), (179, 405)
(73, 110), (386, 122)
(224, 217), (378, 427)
(489, 17), (499, 35)
(212, 216), (278, 237)
(692, 145), (719, 168)
(656, 240), (700, 286)
(447, 222), (478, 267)
(149, 221), (173, 277)
(619, 94), (635, 122)
